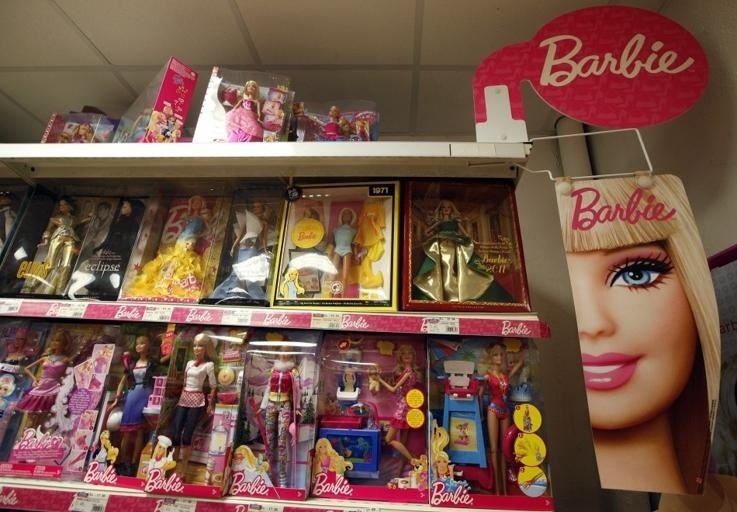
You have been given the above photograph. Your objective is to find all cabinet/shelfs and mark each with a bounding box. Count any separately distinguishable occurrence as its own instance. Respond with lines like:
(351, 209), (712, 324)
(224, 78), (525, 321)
(0, 141), (556, 512)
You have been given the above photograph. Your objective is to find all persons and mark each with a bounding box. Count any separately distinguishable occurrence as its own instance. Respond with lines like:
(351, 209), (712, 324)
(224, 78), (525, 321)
(554, 175), (722, 496)
(59, 81), (353, 143)
(0, 79), (524, 497)
(1, 325), (523, 496)
(1, 190), (495, 300)
